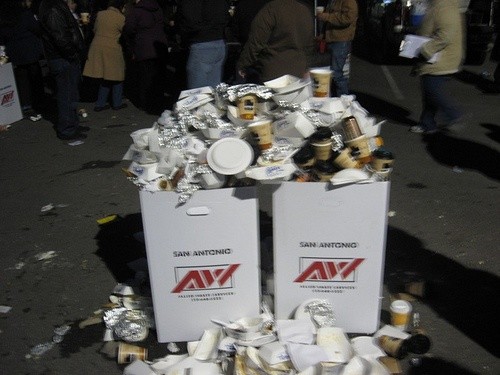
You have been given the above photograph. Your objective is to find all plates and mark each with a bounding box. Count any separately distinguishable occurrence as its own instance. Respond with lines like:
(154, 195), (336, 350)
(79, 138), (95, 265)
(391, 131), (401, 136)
(207, 136), (254, 176)
(264, 74), (312, 94)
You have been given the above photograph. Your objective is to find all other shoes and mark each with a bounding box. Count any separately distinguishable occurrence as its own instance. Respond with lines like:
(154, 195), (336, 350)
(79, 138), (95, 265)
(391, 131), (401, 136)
(410, 125), (438, 134)
(75, 125), (89, 131)
(34, 98), (48, 107)
(135, 96), (145, 108)
(23, 109), (36, 115)
(163, 88), (172, 97)
(56, 133), (86, 140)
(95, 105), (111, 112)
(436, 106), (462, 126)
(112, 104), (128, 109)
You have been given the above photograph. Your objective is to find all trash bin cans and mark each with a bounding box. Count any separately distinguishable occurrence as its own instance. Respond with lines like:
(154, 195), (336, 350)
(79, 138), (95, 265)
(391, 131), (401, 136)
(0, 62), (23, 126)
(271, 176), (391, 335)
(139, 182), (260, 343)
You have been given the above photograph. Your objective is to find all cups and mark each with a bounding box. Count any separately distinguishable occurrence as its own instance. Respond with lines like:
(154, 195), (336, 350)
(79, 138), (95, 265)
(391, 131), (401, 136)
(159, 180), (173, 190)
(236, 94), (256, 120)
(248, 120), (272, 151)
(80, 11), (90, 25)
(316, 6), (324, 13)
(310, 68), (332, 99)
(118, 343), (148, 365)
(368, 146), (395, 171)
(78, 107), (88, 119)
(343, 134), (374, 164)
(0, 55), (8, 65)
(377, 325), (431, 360)
(330, 144), (361, 171)
(390, 300), (413, 332)
(309, 138), (334, 162)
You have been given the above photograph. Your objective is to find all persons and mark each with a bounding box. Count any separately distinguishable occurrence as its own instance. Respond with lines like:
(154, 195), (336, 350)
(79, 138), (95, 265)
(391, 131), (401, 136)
(0, 0), (500, 139)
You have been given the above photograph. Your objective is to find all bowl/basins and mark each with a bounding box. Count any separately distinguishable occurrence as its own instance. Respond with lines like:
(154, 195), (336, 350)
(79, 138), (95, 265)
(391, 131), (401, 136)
(225, 104), (260, 127)
(174, 82), (214, 113)
(245, 346), (267, 373)
(312, 96), (348, 114)
(258, 342), (290, 366)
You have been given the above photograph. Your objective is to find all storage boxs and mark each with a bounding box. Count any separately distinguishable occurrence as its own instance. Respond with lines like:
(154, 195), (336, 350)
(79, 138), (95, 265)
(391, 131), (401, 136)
(271, 181), (392, 333)
(125, 169), (261, 342)
(0, 62), (23, 127)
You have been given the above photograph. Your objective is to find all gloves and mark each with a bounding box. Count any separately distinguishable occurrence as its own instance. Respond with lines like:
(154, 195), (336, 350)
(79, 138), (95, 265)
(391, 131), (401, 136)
(418, 52), (429, 63)
